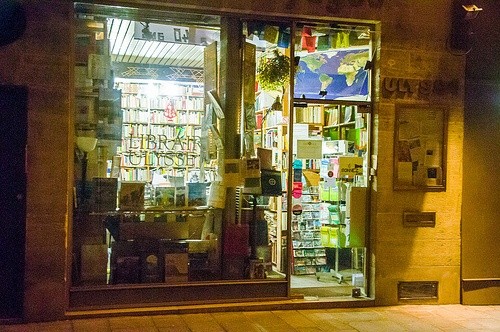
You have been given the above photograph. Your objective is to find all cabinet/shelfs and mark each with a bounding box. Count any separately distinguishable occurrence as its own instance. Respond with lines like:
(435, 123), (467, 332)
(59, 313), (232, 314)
(256, 102), (375, 276)
(115, 72), (206, 184)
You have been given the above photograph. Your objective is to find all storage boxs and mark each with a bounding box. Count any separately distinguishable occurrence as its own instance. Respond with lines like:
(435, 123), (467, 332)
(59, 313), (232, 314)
(92, 178), (118, 211)
(119, 182), (146, 210)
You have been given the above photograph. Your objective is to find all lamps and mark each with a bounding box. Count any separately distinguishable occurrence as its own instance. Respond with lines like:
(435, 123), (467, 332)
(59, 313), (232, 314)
(446, 4), (483, 57)
(75, 136), (99, 207)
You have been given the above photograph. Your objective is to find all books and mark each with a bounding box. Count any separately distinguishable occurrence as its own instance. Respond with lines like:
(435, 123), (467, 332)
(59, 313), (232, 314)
(75, 146), (282, 283)
(76, 15), (120, 143)
(246, 102), (370, 150)
